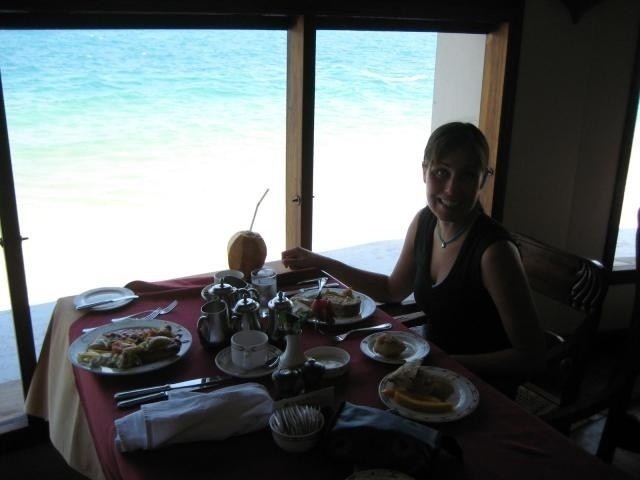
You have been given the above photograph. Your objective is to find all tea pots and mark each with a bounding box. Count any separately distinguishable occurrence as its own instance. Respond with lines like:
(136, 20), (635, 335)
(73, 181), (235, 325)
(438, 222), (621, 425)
(265, 290), (302, 341)
(229, 296), (267, 331)
(209, 279), (237, 306)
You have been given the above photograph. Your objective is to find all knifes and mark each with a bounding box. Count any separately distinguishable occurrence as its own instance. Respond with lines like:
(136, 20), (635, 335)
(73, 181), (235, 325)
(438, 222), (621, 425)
(114, 373), (224, 408)
(116, 383), (218, 408)
(294, 276), (329, 285)
(280, 281), (337, 296)
(75, 294), (140, 312)
(281, 282), (339, 297)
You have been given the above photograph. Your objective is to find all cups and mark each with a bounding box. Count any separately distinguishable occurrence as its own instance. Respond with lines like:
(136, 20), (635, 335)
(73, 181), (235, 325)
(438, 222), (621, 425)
(230, 329), (270, 370)
(213, 269), (244, 283)
(250, 269), (277, 317)
(194, 300), (231, 342)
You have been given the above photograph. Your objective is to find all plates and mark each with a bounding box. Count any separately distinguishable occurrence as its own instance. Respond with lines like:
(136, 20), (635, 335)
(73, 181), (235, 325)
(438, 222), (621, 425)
(214, 345), (284, 379)
(378, 366), (481, 421)
(201, 283), (213, 301)
(73, 286), (135, 313)
(290, 287), (375, 324)
(348, 467), (414, 480)
(359, 330), (431, 363)
(66, 319), (194, 376)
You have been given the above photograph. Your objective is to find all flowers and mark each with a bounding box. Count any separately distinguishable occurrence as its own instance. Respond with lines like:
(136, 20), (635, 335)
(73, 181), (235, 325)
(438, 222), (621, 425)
(273, 293), (338, 332)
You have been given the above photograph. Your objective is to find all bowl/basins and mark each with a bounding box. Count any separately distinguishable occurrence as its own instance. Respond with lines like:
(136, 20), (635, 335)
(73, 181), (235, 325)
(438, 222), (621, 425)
(267, 407), (326, 454)
(304, 346), (352, 377)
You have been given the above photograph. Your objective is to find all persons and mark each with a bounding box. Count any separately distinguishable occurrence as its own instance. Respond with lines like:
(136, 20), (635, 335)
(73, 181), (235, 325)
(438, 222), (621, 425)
(282, 121), (545, 402)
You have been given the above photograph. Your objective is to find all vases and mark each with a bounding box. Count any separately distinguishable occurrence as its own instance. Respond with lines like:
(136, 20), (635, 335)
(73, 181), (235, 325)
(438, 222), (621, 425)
(278, 325), (307, 366)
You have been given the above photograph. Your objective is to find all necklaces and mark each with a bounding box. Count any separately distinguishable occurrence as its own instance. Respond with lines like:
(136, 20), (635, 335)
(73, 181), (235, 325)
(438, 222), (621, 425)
(438, 216), (469, 247)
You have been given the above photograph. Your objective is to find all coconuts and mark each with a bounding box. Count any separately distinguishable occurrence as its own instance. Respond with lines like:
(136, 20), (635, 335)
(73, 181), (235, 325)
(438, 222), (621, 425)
(226, 229), (268, 271)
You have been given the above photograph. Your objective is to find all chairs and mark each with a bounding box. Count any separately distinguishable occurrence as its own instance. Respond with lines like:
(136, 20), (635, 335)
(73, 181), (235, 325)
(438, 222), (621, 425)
(494, 227), (610, 439)
(557, 228), (639, 480)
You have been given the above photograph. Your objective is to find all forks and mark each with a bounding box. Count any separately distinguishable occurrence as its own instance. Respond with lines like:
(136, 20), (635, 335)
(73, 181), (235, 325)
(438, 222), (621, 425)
(329, 322), (391, 342)
(81, 307), (162, 333)
(110, 299), (178, 324)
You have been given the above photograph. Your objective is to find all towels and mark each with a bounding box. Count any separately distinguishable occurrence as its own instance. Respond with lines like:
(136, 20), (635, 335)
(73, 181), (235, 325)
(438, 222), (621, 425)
(112, 382), (277, 453)
